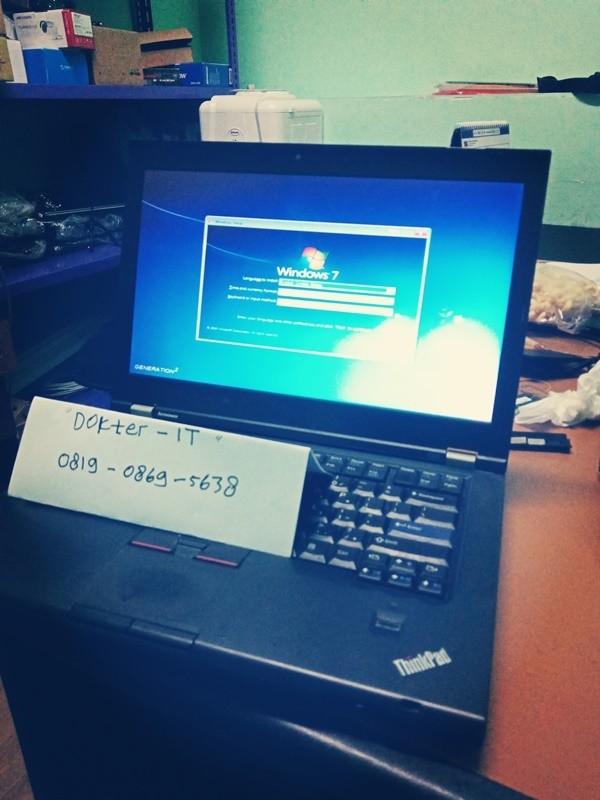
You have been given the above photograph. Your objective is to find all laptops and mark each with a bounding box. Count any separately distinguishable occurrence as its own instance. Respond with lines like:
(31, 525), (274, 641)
(1, 143), (554, 730)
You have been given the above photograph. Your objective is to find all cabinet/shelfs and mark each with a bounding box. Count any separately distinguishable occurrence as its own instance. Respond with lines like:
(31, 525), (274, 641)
(1, 0), (241, 488)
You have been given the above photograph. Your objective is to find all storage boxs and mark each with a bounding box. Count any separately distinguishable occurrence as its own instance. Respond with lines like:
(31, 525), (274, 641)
(1, 5), (233, 87)
